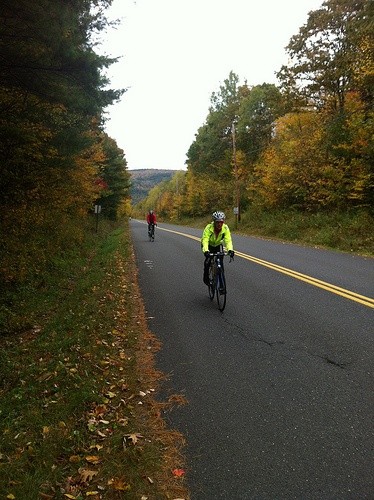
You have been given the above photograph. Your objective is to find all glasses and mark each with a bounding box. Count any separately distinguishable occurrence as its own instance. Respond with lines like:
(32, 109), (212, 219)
(215, 221), (223, 224)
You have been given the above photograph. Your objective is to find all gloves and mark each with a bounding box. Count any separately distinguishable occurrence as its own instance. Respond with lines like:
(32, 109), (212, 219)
(149, 223), (152, 226)
(228, 250), (234, 258)
(204, 252), (210, 258)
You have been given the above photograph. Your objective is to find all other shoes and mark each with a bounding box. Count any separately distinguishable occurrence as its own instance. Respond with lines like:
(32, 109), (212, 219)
(203, 273), (210, 285)
(148, 232), (150, 234)
(219, 289), (226, 295)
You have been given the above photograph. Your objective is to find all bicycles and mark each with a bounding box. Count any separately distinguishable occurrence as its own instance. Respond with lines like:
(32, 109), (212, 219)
(147, 223), (157, 242)
(204, 251), (234, 312)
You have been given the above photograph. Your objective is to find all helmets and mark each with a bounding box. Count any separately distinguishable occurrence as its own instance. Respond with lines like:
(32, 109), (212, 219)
(149, 210), (153, 215)
(212, 211), (225, 220)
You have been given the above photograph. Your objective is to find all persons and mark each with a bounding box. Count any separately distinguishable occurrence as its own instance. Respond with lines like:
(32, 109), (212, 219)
(201, 211), (234, 295)
(146, 210), (156, 239)
